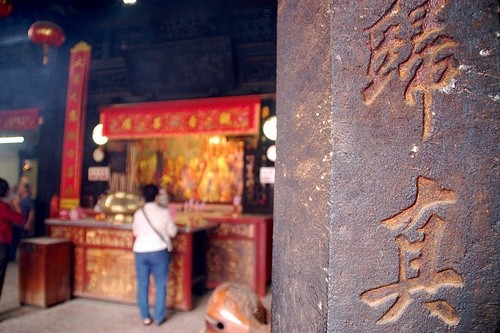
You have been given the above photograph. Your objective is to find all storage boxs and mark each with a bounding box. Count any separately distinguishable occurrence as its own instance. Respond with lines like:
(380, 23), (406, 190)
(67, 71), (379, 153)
(18, 237), (71, 309)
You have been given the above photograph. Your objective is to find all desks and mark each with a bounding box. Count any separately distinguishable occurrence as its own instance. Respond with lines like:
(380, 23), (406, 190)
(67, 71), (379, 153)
(45, 215), (272, 312)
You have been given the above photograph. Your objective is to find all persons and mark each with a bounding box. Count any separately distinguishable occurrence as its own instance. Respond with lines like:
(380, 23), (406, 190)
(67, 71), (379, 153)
(0, 178), (25, 301)
(132, 184), (178, 326)
(8, 183), (34, 262)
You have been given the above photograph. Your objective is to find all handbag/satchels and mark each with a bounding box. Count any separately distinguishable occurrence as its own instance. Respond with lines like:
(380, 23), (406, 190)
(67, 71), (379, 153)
(167, 239), (174, 251)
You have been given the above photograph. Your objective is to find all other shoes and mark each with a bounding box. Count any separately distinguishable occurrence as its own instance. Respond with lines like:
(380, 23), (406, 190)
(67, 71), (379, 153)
(155, 318), (167, 326)
(143, 317), (153, 325)
